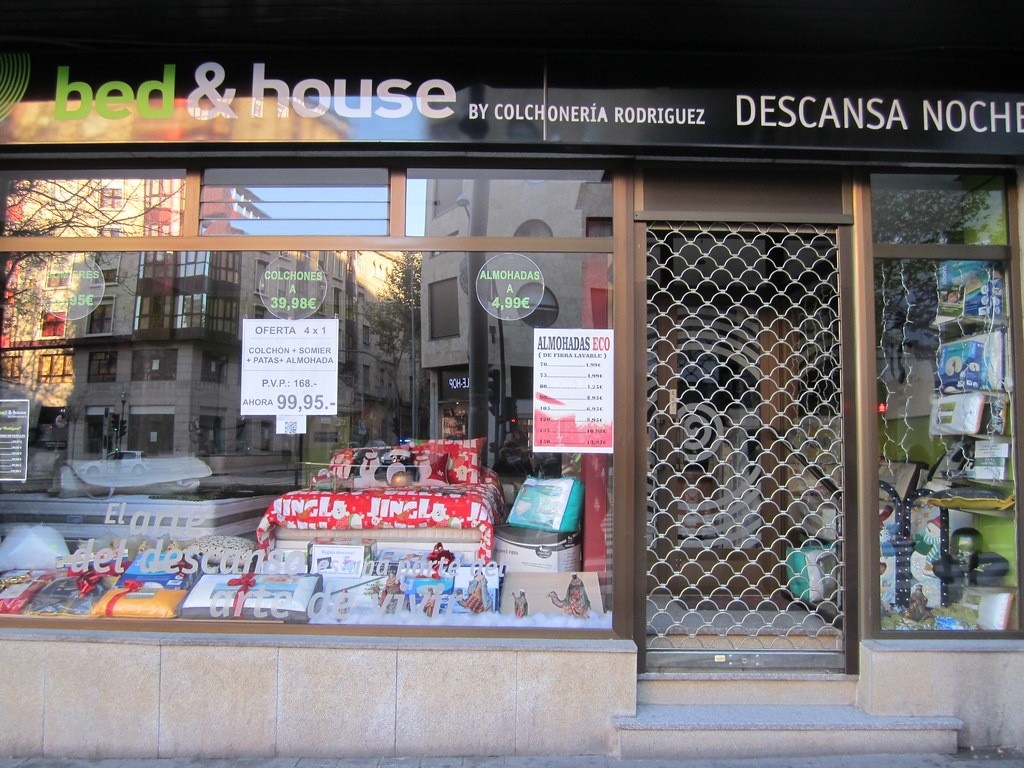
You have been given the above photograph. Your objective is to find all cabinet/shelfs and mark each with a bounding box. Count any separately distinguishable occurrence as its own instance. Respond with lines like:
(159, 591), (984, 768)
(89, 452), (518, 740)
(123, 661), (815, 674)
(924, 311), (1018, 518)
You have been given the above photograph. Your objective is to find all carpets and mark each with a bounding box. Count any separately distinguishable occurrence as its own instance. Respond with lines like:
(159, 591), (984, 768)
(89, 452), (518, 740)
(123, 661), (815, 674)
(643, 595), (838, 637)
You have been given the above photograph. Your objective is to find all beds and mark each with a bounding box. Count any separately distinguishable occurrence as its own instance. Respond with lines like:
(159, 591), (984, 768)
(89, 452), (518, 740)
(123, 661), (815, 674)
(256, 464), (506, 562)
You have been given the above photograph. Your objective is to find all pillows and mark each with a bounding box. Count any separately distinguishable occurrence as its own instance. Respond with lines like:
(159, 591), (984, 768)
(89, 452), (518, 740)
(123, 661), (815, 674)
(328, 444), (411, 478)
(406, 435), (490, 484)
(410, 451), (451, 487)
(346, 448), (396, 488)
(179, 572), (325, 625)
(90, 588), (186, 618)
(180, 535), (258, 567)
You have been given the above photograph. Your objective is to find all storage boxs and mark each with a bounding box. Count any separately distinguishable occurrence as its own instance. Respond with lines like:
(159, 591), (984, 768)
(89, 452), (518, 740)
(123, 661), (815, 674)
(307, 535), (379, 577)
